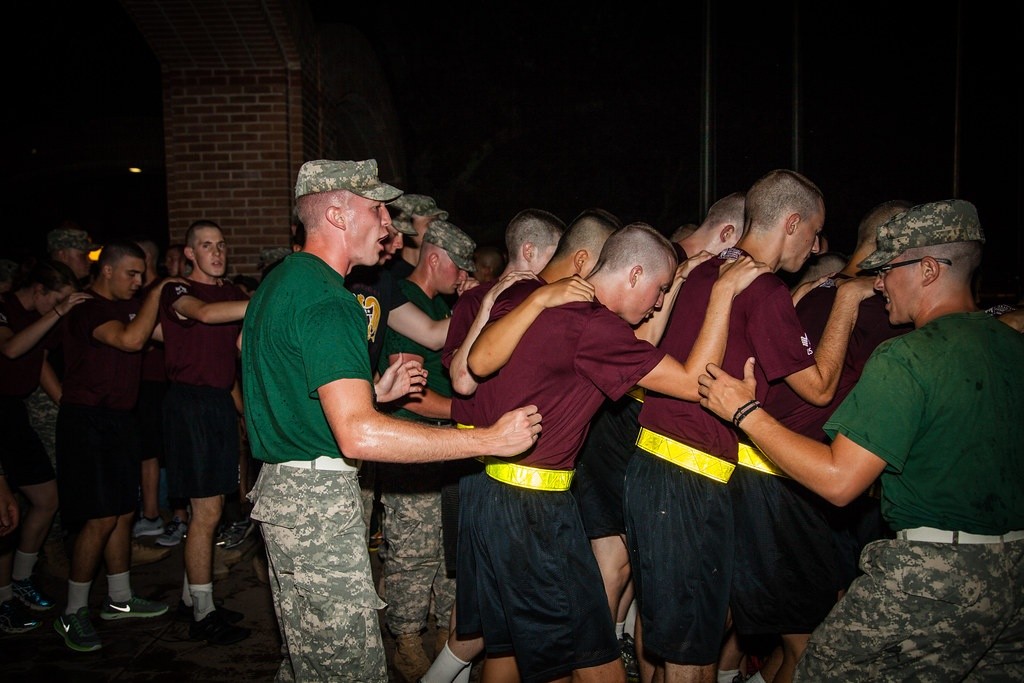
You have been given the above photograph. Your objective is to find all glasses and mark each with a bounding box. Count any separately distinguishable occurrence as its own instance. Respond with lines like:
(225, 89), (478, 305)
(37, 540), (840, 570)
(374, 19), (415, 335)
(855, 259), (951, 286)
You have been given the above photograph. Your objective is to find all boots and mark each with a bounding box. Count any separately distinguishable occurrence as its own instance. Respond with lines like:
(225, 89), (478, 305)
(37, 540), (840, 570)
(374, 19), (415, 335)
(394, 633), (431, 683)
(434, 628), (449, 656)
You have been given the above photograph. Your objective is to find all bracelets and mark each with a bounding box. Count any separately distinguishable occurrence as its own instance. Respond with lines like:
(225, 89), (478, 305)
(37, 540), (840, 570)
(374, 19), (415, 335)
(733, 399), (761, 426)
(53, 307), (62, 317)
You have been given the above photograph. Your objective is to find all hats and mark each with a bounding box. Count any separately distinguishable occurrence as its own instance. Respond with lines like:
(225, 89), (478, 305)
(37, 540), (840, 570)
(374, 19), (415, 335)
(295, 159), (404, 200)
(423, 220), (478, 272)
(257, 247), (293, 269)
(385, 195), (418, 235)
(857, 199), (985, 269)
(48, 229), (100, 249)
(404, 194), (449, 220)
(0, 260), (18, 281)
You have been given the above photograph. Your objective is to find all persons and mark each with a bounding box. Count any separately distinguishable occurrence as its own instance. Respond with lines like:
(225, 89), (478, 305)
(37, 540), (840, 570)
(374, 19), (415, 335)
(0, 219), (270, 651)
(699, 200), (1024, 683)
(345, 194), (771, 683)
(242, 159), (541, 683)
(625, 171), (1024, 683)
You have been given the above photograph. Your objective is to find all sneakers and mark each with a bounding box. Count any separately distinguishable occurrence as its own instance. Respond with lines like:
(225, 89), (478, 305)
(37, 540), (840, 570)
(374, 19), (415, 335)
(11, 581), (54, 610)
(130, 516), (259, 578)
(189, 609), (252, 644)
(101, 595), (169, 619)
(0, 600), (42, 633)
(53, 607), (101, 651)
(176, 598), (244, 622)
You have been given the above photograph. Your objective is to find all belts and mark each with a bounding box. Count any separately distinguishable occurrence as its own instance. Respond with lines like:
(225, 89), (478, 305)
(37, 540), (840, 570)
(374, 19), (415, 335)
(278, 457), (349, 472)
(896, 526), (1024, 544)
